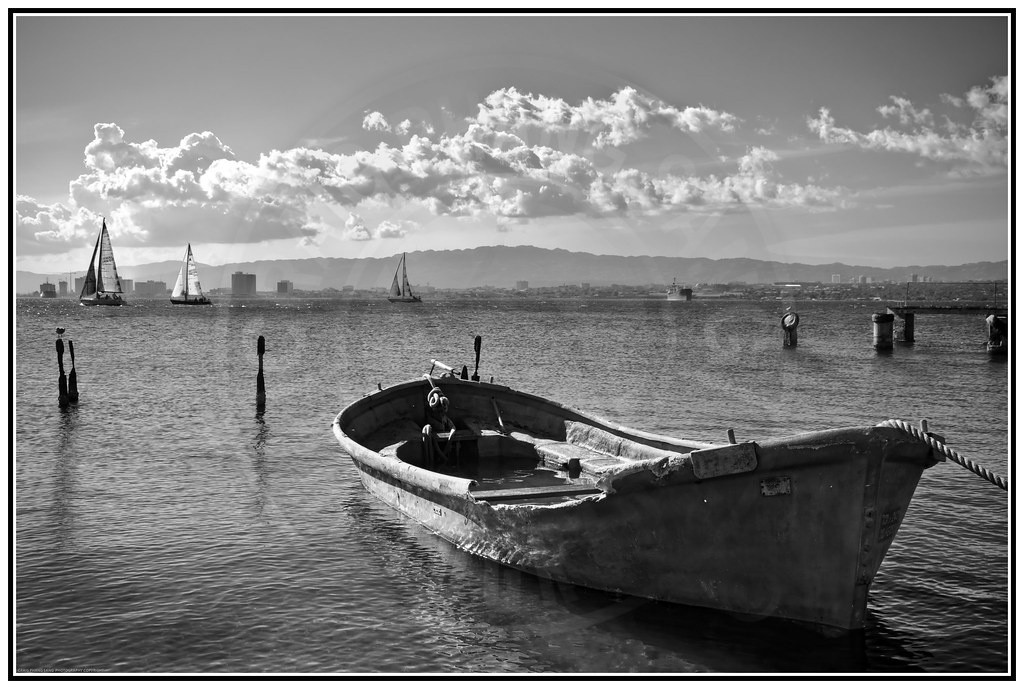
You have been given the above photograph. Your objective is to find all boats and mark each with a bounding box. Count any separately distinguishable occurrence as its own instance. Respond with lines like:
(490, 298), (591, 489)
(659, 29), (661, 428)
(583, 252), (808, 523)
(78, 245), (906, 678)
(666, 276), (693, 302)
(331, 356), (946, 645)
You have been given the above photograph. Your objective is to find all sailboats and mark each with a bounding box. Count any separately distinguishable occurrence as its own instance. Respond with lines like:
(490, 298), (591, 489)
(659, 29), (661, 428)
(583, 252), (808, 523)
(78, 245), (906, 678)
(78, 217), (129, 307)
(169, 243), (214, 307)
(386, 252), (424, 304)
